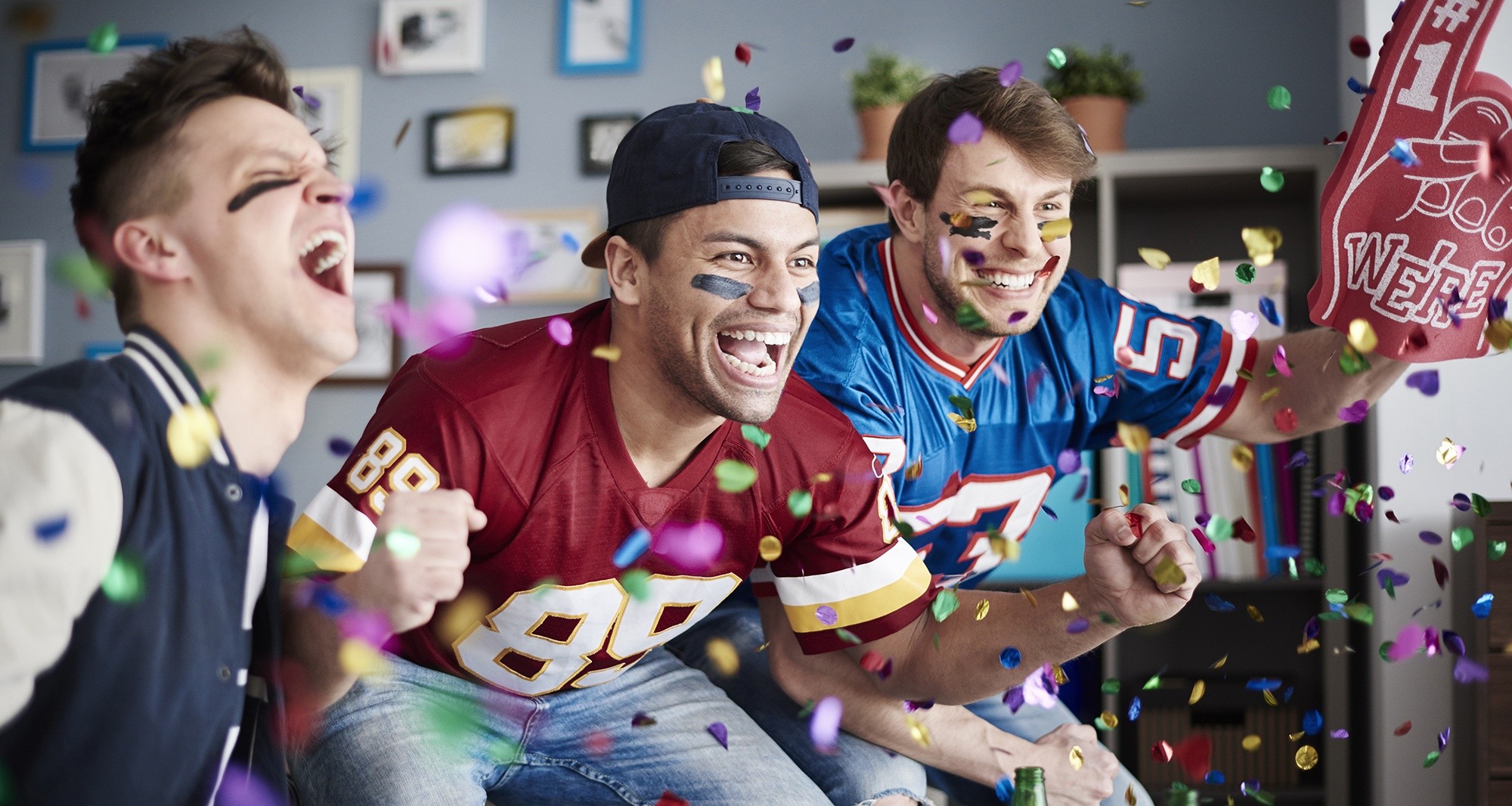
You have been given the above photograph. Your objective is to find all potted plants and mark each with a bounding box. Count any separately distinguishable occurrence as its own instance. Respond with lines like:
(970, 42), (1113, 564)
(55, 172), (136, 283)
(850, 54), (930, 161)
(1043, 41), (1149, 151)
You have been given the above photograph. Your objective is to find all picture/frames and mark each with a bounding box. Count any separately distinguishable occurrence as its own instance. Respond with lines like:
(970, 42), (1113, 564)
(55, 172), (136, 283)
(23, 0), (647, 392)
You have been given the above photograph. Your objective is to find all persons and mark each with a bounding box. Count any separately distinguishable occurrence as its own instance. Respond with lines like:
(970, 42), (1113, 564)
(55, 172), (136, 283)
(0, 25), (359, 806)
(790, 64), (1411, 806)
(273, 99), (1204, 806)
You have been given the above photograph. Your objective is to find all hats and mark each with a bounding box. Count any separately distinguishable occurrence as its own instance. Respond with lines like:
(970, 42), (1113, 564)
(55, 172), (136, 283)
(581, 97), (819, 269)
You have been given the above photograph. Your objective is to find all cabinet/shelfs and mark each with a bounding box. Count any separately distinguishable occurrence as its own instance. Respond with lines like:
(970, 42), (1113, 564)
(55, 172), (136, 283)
(801, 143), (1355, 806)
